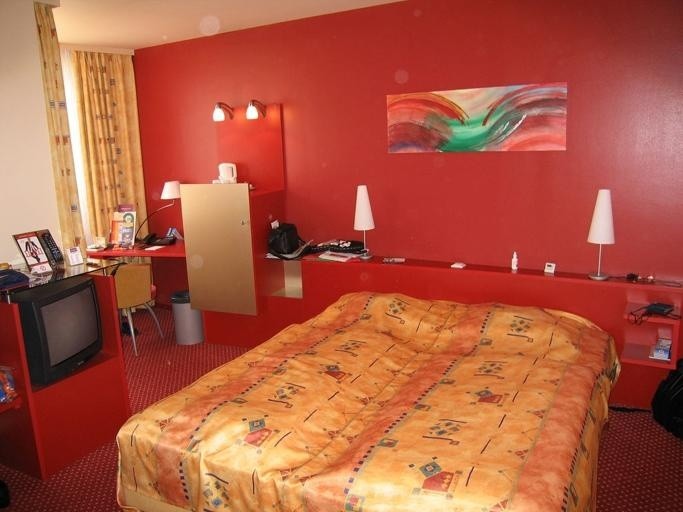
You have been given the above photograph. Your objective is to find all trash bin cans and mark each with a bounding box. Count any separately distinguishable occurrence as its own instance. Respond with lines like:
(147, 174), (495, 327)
(169, 289), (203, 346)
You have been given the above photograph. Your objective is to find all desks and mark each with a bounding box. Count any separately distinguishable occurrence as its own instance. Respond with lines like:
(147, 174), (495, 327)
(85, 239), (185, 259)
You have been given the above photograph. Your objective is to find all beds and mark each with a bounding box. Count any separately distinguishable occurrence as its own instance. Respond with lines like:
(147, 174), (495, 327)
(114, 291), (621, 512)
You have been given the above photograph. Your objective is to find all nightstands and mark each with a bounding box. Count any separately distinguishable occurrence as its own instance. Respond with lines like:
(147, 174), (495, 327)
(0, 273), (132, 479)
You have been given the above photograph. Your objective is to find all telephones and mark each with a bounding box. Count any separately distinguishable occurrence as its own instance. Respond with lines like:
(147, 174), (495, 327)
(138, 232), (176, 249)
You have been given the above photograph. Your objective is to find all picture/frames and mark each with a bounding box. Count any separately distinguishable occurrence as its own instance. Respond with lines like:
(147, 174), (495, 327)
(386, 82), (568, 154)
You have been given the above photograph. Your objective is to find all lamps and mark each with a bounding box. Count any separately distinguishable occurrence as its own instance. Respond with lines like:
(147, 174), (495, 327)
(586, 189), (615, 280)
(245, 98), (266, 121)
(353, 185), (376, 259)
(160, 180), (181, 201)
(212, 102), (234, 122)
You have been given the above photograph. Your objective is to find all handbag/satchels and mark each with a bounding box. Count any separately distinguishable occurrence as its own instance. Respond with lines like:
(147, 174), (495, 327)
(266, 222), (304, 261)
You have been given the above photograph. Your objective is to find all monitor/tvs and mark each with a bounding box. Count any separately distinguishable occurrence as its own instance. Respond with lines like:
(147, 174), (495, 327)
(11, 275), (103, 387)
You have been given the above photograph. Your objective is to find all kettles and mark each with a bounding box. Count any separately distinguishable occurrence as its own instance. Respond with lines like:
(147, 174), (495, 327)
(218, 163), (236, 182)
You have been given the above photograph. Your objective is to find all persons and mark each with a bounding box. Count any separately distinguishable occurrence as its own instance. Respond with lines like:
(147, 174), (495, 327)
(25, 237), (40, 264)
(123, 214), (134, 226)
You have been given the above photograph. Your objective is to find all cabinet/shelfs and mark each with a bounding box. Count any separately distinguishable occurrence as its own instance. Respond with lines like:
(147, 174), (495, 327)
(178, 182), (259, 317)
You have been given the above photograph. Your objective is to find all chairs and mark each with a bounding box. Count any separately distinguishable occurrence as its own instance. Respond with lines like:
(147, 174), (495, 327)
(115, 264), (164, 356)
